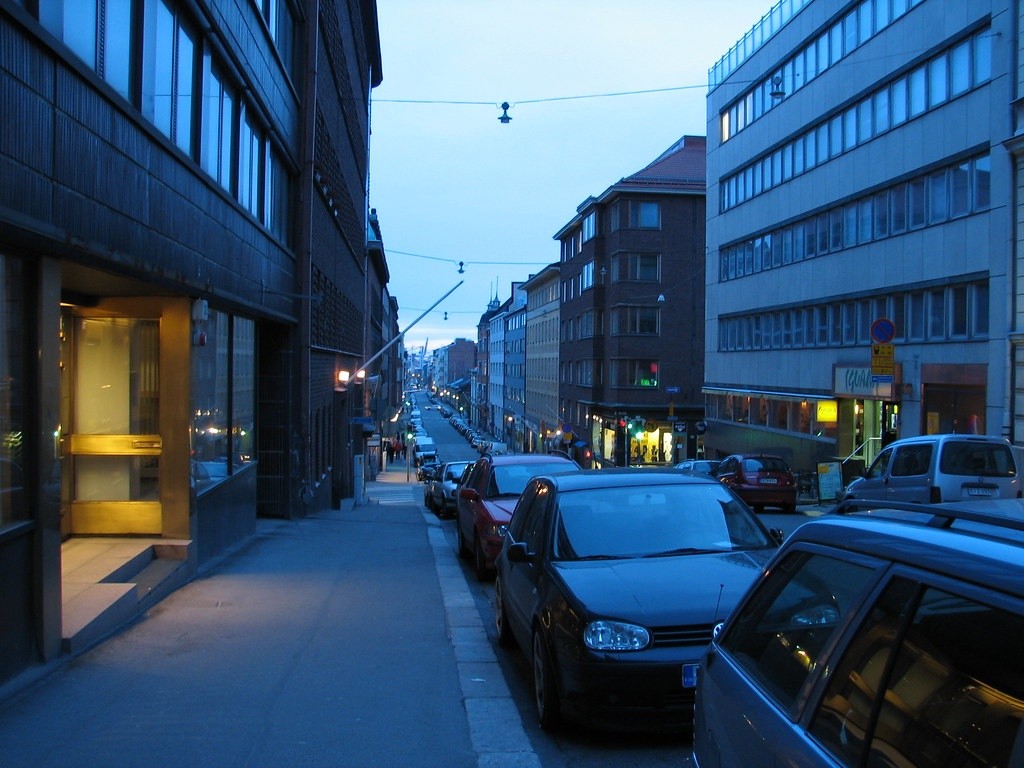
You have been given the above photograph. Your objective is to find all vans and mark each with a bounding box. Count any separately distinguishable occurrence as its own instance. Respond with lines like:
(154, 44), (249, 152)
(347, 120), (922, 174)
(837, 434), (1020, 511)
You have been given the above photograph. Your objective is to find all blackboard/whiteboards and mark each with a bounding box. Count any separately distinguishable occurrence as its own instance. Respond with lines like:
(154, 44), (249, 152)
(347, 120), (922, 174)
(816, 461), (845, 505)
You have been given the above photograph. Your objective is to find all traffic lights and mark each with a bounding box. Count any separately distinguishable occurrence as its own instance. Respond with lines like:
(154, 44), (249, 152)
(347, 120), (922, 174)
(635, 421), (646, 441)
(584, 449), (593, 459)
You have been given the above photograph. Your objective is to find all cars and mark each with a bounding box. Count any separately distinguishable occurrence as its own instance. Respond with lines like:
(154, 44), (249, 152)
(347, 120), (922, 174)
(423, 460), (482, 519)
(710, 453), (798, 516)
(408, 392), (510, 483)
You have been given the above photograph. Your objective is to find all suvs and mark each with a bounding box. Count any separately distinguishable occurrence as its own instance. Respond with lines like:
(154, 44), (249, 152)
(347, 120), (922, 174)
(491, 468), (841, 735)
(456, 449), (589, 582)
(683, 501), (1024, 768)
(671, 459), (722, 483)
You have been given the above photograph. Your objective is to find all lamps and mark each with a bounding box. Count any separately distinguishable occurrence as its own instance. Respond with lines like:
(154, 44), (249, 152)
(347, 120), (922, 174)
(497, 103), (514, 124)
(769, 76), (786, 99)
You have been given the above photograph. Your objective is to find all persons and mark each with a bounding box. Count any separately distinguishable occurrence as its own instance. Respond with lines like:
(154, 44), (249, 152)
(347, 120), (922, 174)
(635, 439), (659, 463)
(468, 418), (472, 426)
(598, 432), (601, 454)
(387, 431), (408, 464)
(401, 405), (416, 414)
